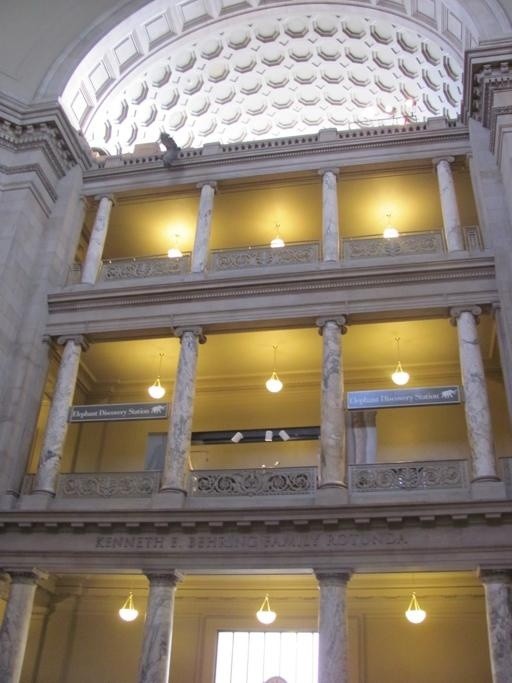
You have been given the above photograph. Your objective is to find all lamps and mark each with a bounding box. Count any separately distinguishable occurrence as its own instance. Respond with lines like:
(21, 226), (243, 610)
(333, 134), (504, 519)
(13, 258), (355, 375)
(265, 345), (286, 396)
(270, 223), (284, 249)
(404, 595), (427, 624)
(230, 429), (245, 443)
(254, 593), (278, 627)
(119, 589), (140, 622)
(265, 429), (290, 443)
(147, 351), (168, 400)
(390, 336), (410, 388)
